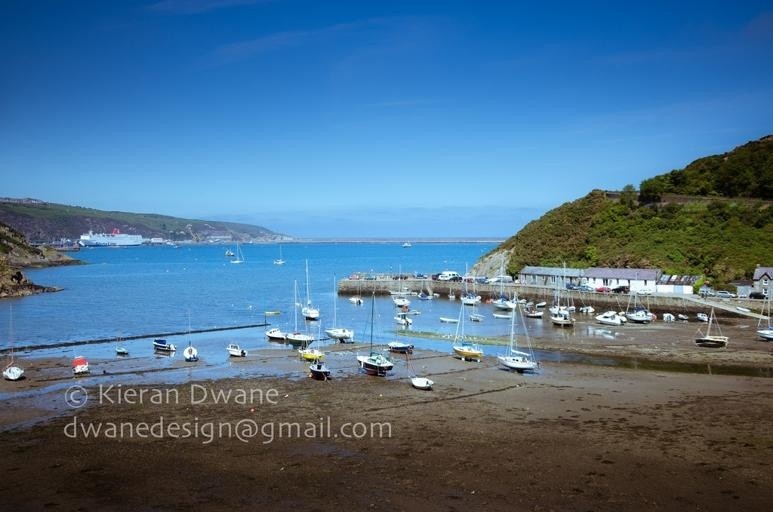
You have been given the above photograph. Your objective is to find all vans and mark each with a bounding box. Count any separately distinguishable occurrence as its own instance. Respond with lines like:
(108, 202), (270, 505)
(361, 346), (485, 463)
(717, 291), (736, 298)
(698, 289), (716, 297)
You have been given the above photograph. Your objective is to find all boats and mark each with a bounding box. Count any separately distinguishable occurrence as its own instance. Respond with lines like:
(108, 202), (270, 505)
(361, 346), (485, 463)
(151, 337), (175, 352)
(153, 349), (175, 360)
(225, 343), (248, 357)
(77, 231), (143, 247)
(411, 376), (434, 390)
(264, 309), (282, 317)
(114, 346), (128, 354)
(71, 354), (89, 373)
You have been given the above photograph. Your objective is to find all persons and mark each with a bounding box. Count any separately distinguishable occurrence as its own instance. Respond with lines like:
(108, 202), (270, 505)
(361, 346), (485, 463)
(402, 305), (408, 320)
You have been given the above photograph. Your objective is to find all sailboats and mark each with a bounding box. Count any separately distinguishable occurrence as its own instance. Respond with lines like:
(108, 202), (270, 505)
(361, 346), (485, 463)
(285, 278), (315, 349)
(2, 302), (24, 382)
(263, 288), (288, 341)
(323, 270), (354, 341)
(300, 257), (319, 320)
(308, 317), (330, 378)
(497, 290), (540, 373)
(182, 309), (198, 362)
(223, 241), (286, 267)
(354, 289), (394, 378)
(692, 306), (730, 350)
(300, 303), (325, 361)
(751, 284), (772, 339)
(450, 298), (482, 363)
(385, 296), (415, 354)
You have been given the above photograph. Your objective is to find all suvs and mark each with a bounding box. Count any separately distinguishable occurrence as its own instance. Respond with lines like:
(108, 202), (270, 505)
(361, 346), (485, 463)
(747, 291), (767, 300)
